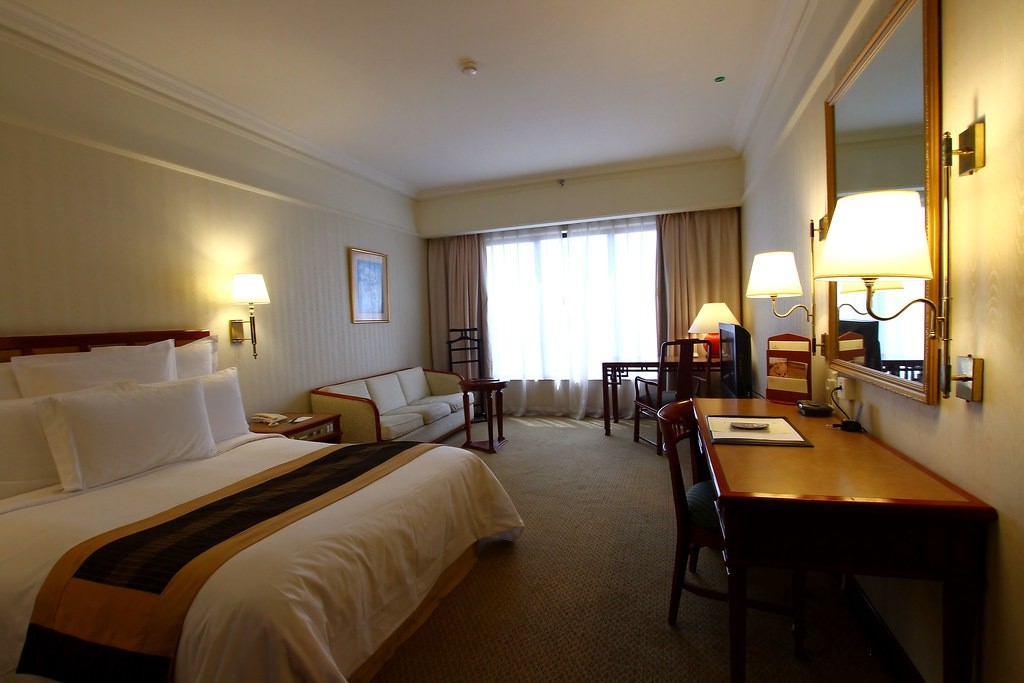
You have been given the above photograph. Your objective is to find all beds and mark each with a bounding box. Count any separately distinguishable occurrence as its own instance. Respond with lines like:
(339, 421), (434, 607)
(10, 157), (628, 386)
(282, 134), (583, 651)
(0, 331), (525, 683)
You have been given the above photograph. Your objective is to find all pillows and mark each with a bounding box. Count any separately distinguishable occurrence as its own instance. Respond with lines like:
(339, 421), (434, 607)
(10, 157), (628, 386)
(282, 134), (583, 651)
(0, 336), (254, 503)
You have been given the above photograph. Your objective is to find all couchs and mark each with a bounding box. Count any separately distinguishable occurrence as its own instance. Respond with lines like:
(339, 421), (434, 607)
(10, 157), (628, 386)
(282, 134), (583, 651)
(310, 366), (475, 443)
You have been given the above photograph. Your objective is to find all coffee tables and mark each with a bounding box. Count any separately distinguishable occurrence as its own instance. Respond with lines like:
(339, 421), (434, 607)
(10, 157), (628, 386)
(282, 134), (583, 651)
(458, 378), (510, 454)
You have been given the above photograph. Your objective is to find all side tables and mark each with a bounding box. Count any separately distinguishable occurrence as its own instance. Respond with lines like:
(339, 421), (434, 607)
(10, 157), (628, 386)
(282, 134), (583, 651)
(249, 412), (344, 444)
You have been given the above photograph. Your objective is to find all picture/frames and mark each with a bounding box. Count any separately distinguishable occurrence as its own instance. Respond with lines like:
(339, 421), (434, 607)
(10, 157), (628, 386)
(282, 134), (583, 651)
(347, 248), (390, 324)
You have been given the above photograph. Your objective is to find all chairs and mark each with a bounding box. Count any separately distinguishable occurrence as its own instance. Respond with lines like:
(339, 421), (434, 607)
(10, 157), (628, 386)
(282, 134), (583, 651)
(634, 339), (712, 455)
(656, 400), (851, 660)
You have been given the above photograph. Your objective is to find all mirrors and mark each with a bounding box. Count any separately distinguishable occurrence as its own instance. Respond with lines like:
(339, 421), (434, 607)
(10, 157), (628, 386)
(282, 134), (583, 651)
(823, 0), (939, 405)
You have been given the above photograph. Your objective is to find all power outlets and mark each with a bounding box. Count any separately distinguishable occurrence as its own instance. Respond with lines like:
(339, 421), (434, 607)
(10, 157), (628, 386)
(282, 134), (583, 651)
(828, 369), (856, 400)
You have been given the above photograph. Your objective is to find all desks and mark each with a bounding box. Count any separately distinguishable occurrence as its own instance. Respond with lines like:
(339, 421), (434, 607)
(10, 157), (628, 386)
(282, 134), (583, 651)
(692, 397), (998, 683)
(602, 359), (721, 436)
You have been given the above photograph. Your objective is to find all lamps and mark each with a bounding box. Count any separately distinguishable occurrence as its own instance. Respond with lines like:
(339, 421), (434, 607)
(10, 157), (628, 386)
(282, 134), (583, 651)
(229, 274), (271, 359)
(837, 280), (904, 359)
(688, 302), (741, 359)
(746, 115), (985, 403)
(461, 60), (478, 76)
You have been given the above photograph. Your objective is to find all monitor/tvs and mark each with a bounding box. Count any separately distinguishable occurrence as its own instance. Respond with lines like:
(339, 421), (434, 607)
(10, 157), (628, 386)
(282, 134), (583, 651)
(718, 322), (753, 400)
